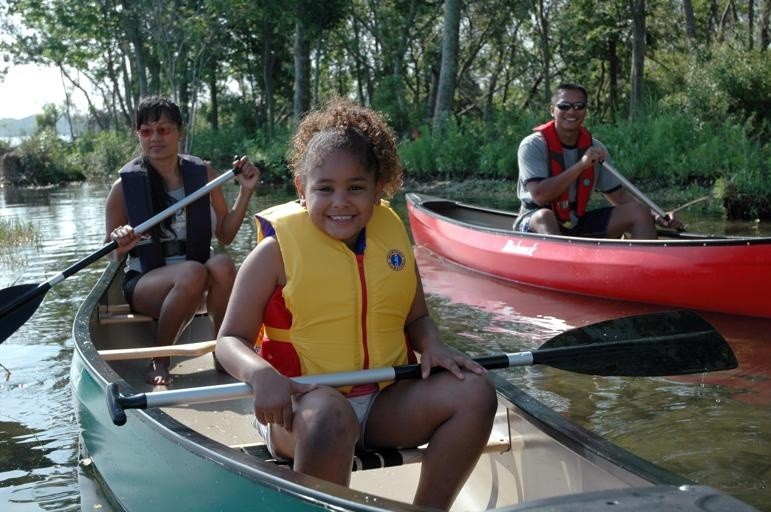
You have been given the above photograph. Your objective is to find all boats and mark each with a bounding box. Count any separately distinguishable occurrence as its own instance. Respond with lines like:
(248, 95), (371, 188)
(405, 191), (771, 320)
(69, 260), (766, 512)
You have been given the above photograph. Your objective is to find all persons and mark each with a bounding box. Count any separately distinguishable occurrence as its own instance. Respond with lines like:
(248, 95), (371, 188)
(512, 84), (684, 239)
(214, 93), (498, 512)
(104, 96), (260, 386)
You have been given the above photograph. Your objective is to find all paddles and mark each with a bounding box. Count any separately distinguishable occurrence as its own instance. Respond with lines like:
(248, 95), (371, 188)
(0, 152), (243, 347)
(106, 309), (741, 426)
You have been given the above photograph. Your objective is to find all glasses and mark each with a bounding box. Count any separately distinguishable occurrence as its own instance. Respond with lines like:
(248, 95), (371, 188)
(139, 125), (178, 137)
(552, 101), (586, 111)
